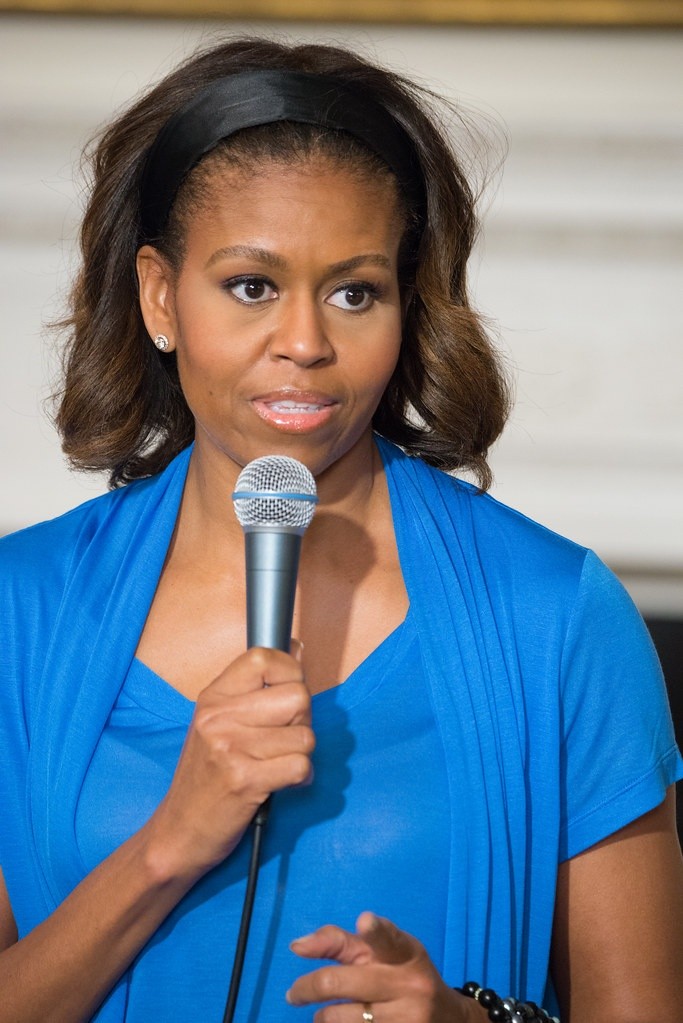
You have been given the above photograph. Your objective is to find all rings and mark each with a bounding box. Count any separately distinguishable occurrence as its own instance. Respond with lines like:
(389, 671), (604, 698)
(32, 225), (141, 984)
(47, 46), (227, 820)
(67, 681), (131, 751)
(360, 1002), (372, 1022)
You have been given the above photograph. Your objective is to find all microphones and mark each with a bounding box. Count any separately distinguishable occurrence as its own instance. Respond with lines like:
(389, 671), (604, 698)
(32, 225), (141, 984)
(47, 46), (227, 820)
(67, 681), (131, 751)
(233, 457), (319, 820)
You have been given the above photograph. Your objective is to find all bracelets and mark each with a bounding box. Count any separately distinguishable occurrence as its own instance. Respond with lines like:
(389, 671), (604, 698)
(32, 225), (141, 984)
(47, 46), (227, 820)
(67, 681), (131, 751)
(460, 979), (565, 1023)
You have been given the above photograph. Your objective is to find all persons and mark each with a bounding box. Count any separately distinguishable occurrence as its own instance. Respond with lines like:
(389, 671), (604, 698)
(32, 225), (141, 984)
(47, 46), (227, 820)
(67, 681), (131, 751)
(0, 36), (683, 1023)
